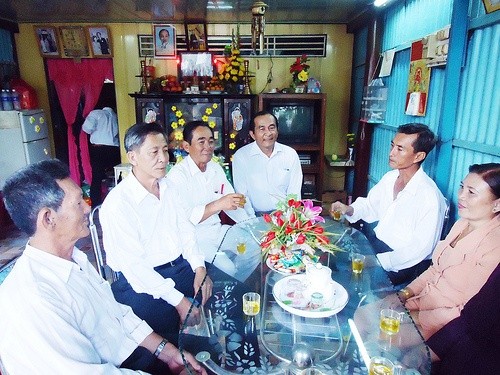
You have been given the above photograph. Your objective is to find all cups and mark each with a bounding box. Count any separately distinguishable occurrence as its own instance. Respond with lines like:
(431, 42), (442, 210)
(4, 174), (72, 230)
(331, 211), (341, 220)
(331, 153), (337, 160)
(243, 292), (261, 315)
(369, 356), (396, 375)
(380, 309), (401, 335)
(352, 253), (365, 273)
(186, 86), (198, 92)
(238, 194), (247, 207)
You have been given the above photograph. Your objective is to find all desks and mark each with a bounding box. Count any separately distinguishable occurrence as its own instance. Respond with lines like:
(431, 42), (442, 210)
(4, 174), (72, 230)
(323, 154), (354, 167)
(176, 215), (433, 375)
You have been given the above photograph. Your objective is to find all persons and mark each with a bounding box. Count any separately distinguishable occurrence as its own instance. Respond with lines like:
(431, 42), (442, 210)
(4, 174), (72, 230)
(331, 123), (447, 318)
(231, 110), (303, 222)
(351, 163), (500, 358)
(99, 121), (261, 370)
(0, 158), (207, 375)
(392, 262), (500, 375)
(164, 119), (263, 283)
(158, 29), (173, 54)
(92, 32), (110, 55)
(82, 95), (121, 211)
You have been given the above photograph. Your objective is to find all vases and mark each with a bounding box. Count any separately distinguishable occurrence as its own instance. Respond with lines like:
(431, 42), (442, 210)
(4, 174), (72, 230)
(347, 148), (353, 162)
(229, 81), (244, 94)
(293, 82), (306, 94)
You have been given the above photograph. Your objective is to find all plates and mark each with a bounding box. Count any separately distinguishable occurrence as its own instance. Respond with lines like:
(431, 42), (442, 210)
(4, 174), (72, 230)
(209, 91), (221, 94)
(273, 273), (349, 318)
(160, 89), (183, 93)
(265, 257), (332, 275)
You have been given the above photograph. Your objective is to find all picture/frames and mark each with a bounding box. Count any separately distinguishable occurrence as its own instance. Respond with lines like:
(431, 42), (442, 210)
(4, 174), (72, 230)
(151, 24), (178, 61)
(85, 25), (115, 58)
(183, 21), (209, 53)
(32, 25), (62, 57)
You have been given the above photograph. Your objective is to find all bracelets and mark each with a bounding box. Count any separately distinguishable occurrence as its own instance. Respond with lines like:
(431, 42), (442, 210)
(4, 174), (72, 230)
(400, 289), (409, 298)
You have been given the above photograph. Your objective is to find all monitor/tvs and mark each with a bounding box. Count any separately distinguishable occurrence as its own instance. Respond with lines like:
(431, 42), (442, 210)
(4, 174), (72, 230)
(270, 101), (318, 144)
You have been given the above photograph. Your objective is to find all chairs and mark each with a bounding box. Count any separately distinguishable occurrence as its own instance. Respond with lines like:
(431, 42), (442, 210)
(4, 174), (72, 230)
(87, 204), (111, 283)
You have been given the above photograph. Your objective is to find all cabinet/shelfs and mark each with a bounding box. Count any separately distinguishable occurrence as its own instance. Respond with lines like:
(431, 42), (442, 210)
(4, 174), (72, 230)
(128, 93), (259, 181)
(259, 93), (328, 210)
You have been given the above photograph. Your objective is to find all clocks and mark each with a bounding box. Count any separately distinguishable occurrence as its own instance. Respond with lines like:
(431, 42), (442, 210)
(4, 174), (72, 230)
(56, 26), (93, 60)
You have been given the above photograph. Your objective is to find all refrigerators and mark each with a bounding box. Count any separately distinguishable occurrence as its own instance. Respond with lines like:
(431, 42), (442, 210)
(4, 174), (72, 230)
(0, 109), (52, 191)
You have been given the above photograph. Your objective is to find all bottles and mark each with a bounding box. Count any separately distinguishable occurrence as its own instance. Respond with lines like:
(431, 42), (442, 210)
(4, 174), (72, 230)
(11, 89), (21, 110)
(0, 89), (13, 110)
(193, 39), (204, 50)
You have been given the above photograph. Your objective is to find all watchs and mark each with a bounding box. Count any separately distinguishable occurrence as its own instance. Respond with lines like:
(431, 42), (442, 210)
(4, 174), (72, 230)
(153, 338), (168, 357)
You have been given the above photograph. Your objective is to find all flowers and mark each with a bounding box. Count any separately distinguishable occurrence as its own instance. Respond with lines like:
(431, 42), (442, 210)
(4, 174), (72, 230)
(218, 50), (246, 83)
(245, 190), (349, 268)
(289, 53), (311, 84)
(347, 133), (355, 148)
